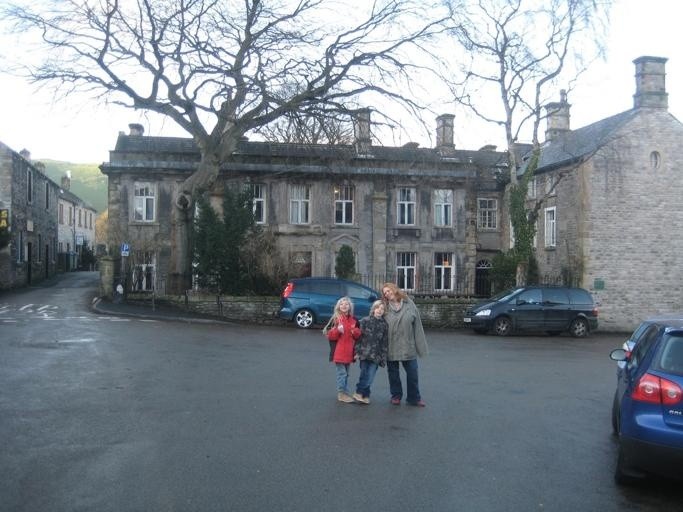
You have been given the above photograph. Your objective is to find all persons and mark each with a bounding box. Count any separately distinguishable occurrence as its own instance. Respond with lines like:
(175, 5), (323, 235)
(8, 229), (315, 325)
(381, 282), (428, 407)
(80, 245), (107, 272)
(352, 300), (389, 404)
(323, 296), (362, 403)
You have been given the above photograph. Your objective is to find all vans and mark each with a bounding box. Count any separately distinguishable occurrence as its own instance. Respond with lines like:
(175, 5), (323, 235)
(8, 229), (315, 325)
(278, 275), (382, 328)
(462, 285), (599, 337)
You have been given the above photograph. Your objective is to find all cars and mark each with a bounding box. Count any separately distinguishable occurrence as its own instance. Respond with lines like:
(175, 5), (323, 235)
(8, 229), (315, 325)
(616, 318), (683, 380)
(609, 321), (683, 485)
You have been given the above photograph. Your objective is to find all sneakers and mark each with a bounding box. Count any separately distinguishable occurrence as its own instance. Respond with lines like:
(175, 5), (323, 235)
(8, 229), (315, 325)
(407, 401), (425, 406)
(338, 392), (370, 403)
(391, 397), (400, 404)
